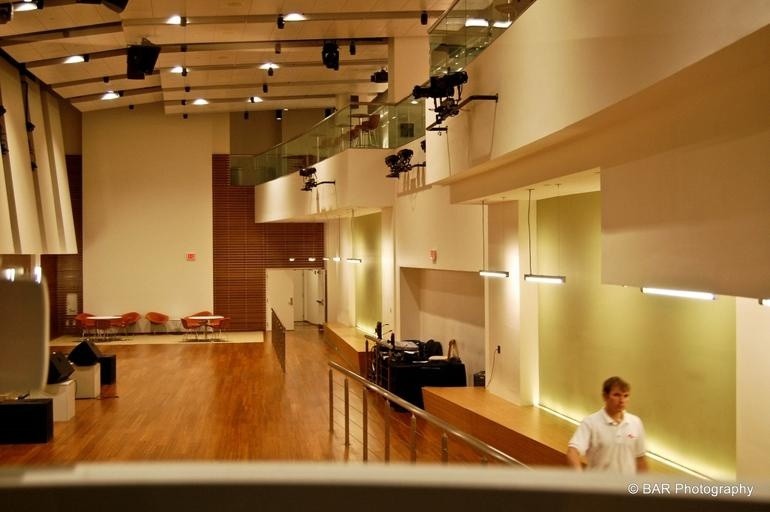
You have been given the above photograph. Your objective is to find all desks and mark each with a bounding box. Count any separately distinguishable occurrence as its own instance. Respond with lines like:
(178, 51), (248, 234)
(190, 316), (224, 342)
(86, 317), (122, 342)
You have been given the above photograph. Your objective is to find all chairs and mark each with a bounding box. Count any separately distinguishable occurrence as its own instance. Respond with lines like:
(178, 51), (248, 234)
(94, 320), (112, 341)
(321, 114), (380, 155)
(181, 319), (201, 342)
(146, 312), (170, 337)
(123, 313), (141, 339)
(110, 319), (122, 341)
(191, 311), (211, 339)
(76, 314), (95, 341)
(207, 317), (231, 342)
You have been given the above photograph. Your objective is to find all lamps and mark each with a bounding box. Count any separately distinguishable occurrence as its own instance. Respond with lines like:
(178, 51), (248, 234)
(480, 198), (511, 278)
(413, 73), (500, 136)
(524, 187), (566, 288)
(126, 45), (161, 80)
(322, 41), (339, 71)
(384, 139), (426, 177)
(75, 1), (128, 13)
(346, 210), (362, 263)
(640, 288), (717, 301)
(332, 216), (342, 262)
(300, 169), (335, 191)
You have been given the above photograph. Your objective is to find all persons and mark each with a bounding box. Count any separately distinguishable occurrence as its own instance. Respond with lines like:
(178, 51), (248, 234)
(569, 377), (650, 476)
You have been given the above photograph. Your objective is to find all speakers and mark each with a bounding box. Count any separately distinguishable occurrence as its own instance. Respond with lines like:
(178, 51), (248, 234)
(0, 398), (54, 444)
(101, 355), (116, 384)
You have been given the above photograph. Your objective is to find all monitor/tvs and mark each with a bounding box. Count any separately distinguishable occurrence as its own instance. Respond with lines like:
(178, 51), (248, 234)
(375, 321), (383, 339)
(391, 333), (395, 350)
